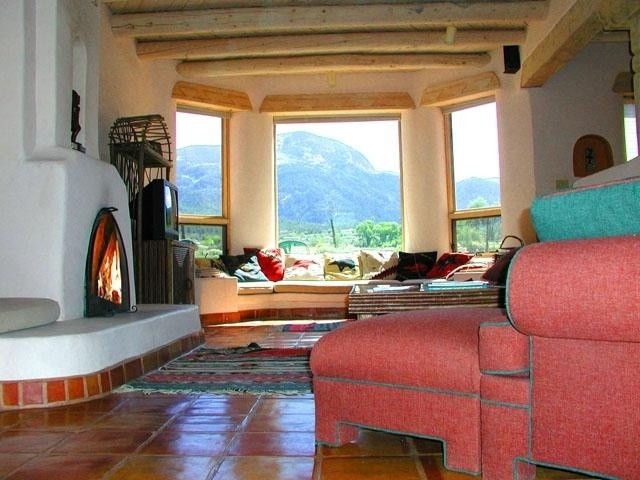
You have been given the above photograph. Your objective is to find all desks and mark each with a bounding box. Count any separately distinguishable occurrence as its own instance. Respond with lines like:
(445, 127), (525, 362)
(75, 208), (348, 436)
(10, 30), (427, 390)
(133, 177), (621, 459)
(348, 282), (504, 320)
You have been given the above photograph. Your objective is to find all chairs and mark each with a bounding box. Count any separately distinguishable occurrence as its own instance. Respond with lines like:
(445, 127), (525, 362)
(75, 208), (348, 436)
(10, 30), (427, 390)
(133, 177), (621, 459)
(309, 157), (640, 480)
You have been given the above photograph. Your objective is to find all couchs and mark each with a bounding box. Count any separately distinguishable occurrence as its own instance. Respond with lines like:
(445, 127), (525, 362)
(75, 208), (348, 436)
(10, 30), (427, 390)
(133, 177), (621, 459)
(194, 250), (515, 326)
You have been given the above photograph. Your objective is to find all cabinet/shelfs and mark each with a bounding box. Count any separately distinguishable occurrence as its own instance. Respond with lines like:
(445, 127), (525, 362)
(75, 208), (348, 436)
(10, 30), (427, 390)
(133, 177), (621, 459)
(105, 114), (177, 303)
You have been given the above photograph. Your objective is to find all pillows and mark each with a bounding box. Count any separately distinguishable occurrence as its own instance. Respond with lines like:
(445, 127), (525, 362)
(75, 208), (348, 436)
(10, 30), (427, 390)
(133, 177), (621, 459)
(213, 248), (492, 282)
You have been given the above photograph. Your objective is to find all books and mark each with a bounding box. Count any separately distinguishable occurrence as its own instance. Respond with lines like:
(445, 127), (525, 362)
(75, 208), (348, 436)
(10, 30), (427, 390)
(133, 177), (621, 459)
(425, 261), (491, 290)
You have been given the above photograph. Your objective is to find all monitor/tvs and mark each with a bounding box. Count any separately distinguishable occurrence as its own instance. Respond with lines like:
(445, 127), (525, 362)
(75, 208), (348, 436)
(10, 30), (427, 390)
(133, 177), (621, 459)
(131, 178), (178, 240)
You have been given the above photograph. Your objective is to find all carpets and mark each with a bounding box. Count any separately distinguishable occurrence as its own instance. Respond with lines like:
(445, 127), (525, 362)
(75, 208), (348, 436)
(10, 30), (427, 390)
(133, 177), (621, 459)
(277, 321), (348, 333)
(111, 344), (316, 396)
